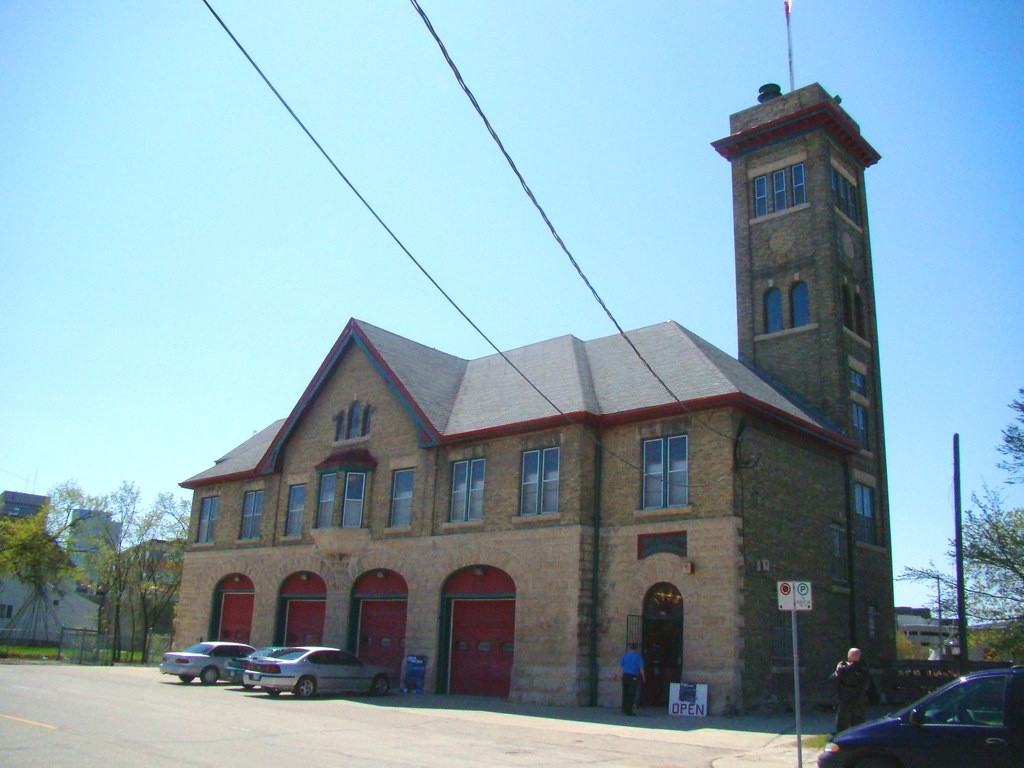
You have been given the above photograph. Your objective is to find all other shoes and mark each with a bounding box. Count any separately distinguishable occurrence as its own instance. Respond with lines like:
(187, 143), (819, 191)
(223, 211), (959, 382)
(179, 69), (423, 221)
(627, 712), (636, 717)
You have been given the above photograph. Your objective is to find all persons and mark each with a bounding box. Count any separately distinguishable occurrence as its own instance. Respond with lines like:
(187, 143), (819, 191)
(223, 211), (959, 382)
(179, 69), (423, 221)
(828, 646), (870, 732)
(611, 642), (647, 717)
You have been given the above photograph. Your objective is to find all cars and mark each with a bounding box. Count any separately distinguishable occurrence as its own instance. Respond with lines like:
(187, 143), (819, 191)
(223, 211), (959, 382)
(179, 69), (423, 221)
(243, 645), (390, 699)
(817, 665), (1024, 768)
(158, 642), (259, 684)
(222, 647), (289, 690)
